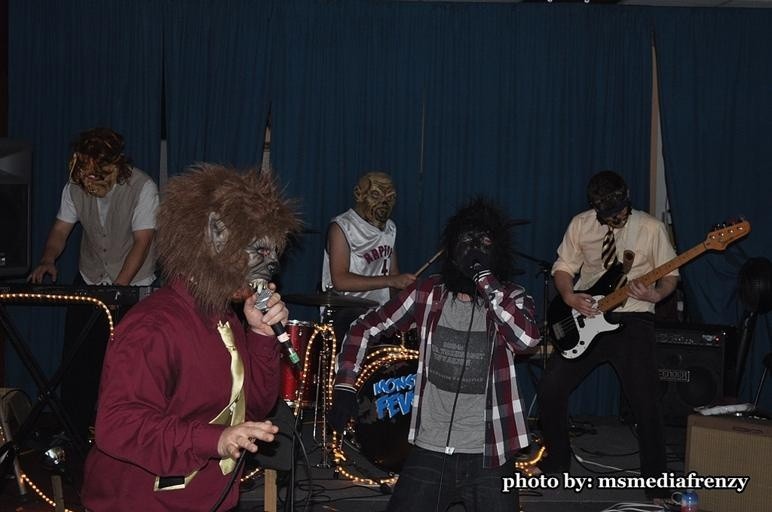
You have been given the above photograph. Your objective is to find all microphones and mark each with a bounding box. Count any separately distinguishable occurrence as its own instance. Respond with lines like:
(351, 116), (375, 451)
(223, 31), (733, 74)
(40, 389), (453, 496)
(253, 290), (300, 365)
(468, 248), (482, 272)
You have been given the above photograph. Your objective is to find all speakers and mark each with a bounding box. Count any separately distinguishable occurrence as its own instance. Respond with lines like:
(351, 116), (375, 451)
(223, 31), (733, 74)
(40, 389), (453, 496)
(618, 323), (738, 428)
(0, 137), (32, 281)
(685, 409), (772, 512)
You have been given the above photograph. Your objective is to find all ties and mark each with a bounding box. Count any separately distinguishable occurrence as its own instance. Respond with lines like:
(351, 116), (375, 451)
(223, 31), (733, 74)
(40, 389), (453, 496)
(602, 218), (629, 308)
(153, 320), (247, 492)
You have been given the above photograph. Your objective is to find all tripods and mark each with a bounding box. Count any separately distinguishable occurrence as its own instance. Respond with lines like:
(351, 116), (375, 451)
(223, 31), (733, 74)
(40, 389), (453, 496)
(514, 249), (576, 438)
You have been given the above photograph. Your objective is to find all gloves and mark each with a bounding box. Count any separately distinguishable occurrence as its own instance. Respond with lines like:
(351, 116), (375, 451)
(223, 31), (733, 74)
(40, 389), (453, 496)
(326, 385), (362, 440)
(465, 259), (490, 279)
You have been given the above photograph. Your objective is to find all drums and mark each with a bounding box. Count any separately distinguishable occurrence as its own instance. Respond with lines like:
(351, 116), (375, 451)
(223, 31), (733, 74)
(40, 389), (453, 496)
(280, 317), (318, 411)
(352, 343), (420, 475)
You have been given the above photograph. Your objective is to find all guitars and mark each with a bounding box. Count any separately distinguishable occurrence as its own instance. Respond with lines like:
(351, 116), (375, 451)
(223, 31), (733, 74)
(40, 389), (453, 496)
(545, 217), (750, 363)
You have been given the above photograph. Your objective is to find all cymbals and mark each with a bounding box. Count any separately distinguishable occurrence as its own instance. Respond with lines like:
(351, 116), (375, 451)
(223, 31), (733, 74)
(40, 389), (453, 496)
(278, 290), (380, 309)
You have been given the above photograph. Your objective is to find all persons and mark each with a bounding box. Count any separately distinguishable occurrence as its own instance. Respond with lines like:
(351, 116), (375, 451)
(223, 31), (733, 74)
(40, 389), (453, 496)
(539, 171), (681, 476)
(319, 169), (416, 306)
(326, 190), (542, 510)
(26, 124), (162, 285)
(81, 163), (303, 512)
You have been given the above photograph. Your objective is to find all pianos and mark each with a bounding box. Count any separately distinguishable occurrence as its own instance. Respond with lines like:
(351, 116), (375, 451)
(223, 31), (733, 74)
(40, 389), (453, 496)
(0, 281), (140, 312)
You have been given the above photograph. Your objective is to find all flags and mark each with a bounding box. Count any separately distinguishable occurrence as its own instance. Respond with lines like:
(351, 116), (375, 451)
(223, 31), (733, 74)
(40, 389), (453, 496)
(258, 90), (274, 176)
(648, 46), (676, 248)
(156, 33), (168, 194)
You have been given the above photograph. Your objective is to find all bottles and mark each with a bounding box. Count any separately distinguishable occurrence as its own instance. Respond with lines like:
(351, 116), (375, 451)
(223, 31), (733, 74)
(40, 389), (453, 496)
(43, 432), (68, 463)
